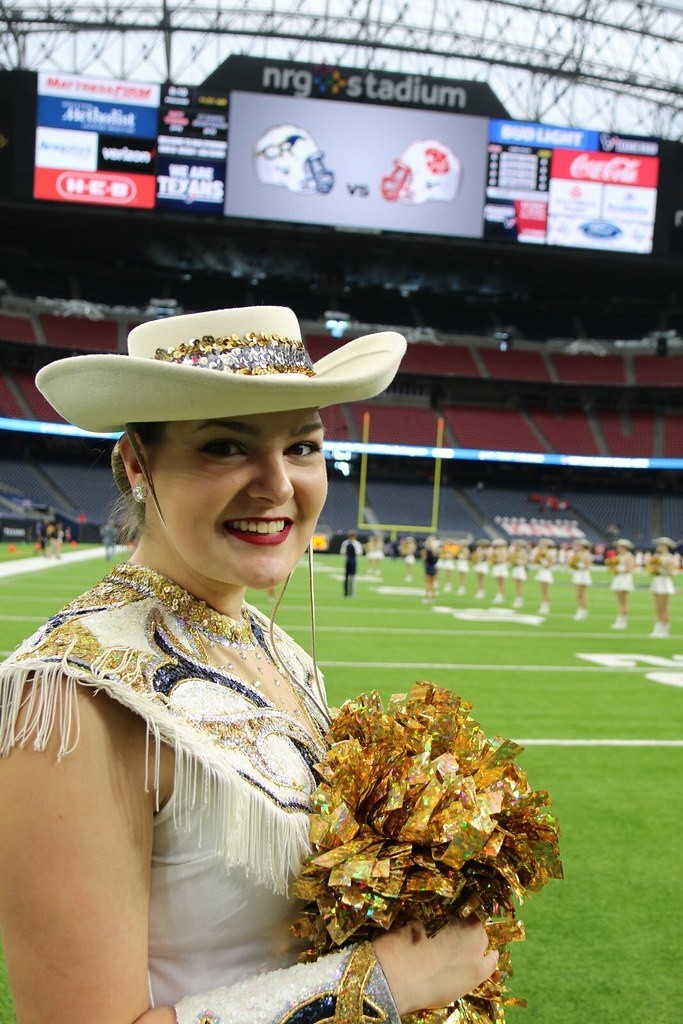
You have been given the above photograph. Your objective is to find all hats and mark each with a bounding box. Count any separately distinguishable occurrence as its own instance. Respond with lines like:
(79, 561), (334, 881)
(31, 303), (409, 438)
(613, 540), (635, 549)
(539, 536), (557, 548)
(491, 537), (509, 546)
(512, 537), (528, 545)
(655, 533), (676, 548)
(576, 539), (591, 547)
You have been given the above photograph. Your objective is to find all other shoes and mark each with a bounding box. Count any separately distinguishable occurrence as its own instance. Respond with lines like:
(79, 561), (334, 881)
(650, 621), (671, 638)
(612, 614), (627, 629)
(511, 596), (523, 607)
(457, 585), (467, 595)
(444, 582), (452, 592)
(492, 592), (506, 603)
(574, 607), (588, 621)
(538, 600), (550, 614)
(475, 588), (484, 597)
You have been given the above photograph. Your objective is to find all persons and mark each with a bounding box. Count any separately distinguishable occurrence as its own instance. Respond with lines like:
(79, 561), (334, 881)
(568, 539), (593, 620)
(534, 538), (555, 614)
(490, 539), (508, 604)
(36, 518), (64, 560)
(340, 529), (363, 597)
(605, 539), (636, 629)
(365, 536), (385, 576)
(0, 306), (500, 1024)
(423, 535), (440, 603)
(645, 537), (676, 638)
(509, 539), (528, 607)
(472, 539), (493, 598)
(443, 539), (455, 592)
(399, 536), (416, 582)
(457, 539), (470, 594)
(100, 519), (117, 560)
